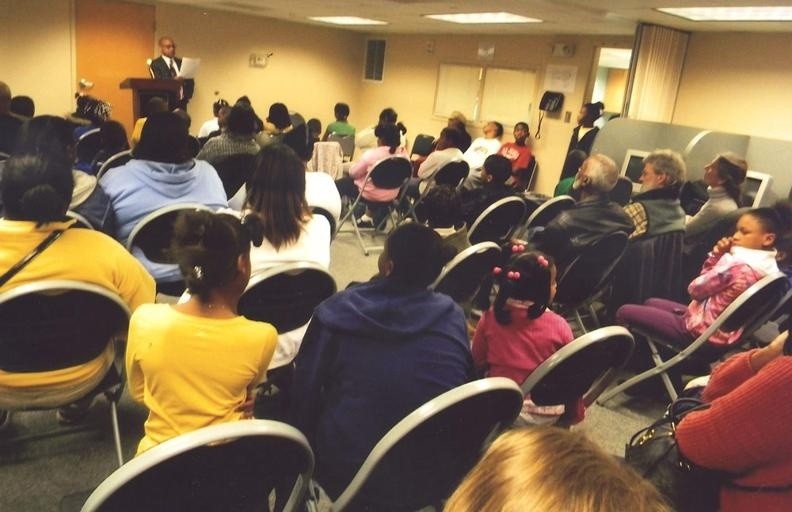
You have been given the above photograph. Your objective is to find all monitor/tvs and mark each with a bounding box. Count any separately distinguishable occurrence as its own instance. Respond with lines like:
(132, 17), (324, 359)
(620, 147), (650, 194)
(736, 170), (773, 210)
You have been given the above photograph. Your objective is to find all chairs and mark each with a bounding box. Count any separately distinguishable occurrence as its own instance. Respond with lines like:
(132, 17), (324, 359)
(80, 419), (315, 512)
(321, 377), (523, 512)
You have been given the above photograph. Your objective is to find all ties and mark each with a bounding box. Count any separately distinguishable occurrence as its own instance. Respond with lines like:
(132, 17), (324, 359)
(169, 60), (177, 78)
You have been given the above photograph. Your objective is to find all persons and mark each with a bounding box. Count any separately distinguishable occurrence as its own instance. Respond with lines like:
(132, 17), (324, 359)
(0, 82), (792, 511)
(151, 37), (195, 110)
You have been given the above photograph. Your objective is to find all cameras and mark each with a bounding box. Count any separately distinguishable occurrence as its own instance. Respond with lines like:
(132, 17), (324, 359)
(79, 77), (94, 89)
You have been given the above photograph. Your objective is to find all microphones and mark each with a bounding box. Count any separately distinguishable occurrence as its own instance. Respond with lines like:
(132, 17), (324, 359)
(147, 58), (152, 70)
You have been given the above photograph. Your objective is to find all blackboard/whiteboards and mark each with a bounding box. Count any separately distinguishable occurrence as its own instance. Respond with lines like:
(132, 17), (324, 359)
(431, 61), (541, 138)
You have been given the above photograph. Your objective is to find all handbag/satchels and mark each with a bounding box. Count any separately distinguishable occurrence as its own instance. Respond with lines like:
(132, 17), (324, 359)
(625, 385), (720, 512)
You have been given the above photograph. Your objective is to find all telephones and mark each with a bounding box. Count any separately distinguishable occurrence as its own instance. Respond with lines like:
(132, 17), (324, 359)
(534, 91), (564, 113)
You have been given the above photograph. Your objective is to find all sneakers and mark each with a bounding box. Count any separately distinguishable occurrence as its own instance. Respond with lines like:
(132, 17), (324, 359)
(56, 394), (96, 424)
(0, 407), (11, 432)
(355, 214), (374, 228)
